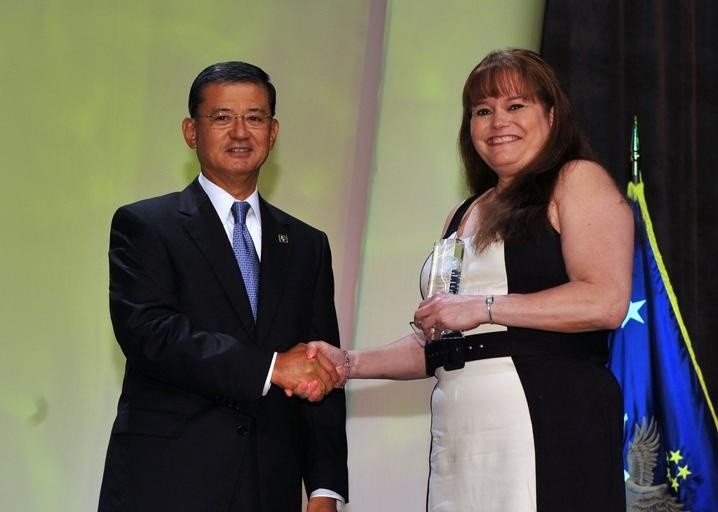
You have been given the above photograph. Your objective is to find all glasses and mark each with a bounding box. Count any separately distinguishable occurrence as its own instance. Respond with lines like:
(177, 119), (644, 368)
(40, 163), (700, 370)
(202, 113), (272, 128)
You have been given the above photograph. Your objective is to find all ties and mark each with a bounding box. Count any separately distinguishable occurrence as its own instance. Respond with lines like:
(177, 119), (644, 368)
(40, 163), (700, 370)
(231, 201), (261, 325)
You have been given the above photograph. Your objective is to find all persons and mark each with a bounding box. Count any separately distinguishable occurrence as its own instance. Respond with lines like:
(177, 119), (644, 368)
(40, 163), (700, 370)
(283, 46), (636, 512)
(95, 63), (352, 512)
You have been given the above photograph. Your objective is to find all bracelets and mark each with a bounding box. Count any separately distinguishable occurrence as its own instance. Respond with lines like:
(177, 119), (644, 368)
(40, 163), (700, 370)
(484, 295), (495, 324)
(338, 348), (351, 388)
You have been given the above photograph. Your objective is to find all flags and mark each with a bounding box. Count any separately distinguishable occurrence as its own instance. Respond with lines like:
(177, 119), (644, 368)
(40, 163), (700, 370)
(602, 179), (718, 512)
